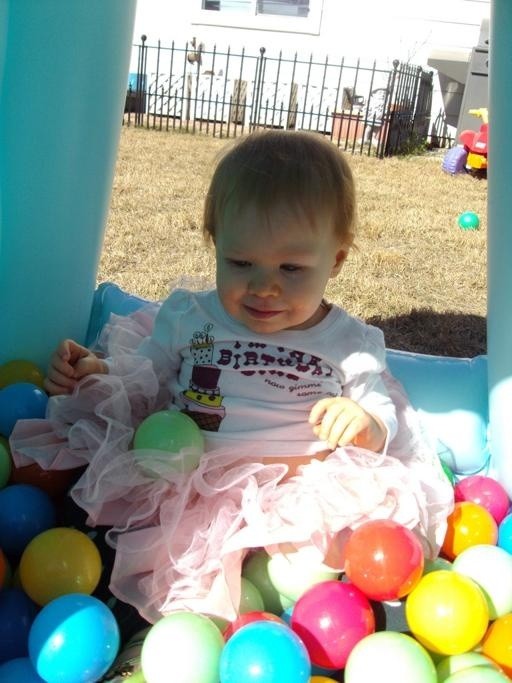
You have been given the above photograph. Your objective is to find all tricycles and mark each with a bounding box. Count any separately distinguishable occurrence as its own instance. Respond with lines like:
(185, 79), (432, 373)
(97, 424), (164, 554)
(441, 107), (489, 176)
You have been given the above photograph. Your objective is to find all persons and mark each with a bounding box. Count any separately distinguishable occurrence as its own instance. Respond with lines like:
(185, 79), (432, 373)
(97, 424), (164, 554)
(41, 131), (456, 627)
